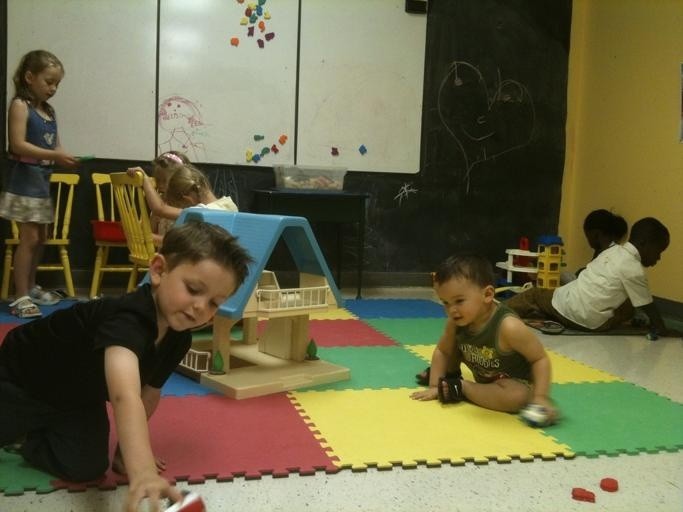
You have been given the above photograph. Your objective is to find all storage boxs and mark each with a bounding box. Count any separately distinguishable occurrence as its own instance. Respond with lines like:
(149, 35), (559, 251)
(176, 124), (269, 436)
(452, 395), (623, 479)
(272, 161), (349, 192)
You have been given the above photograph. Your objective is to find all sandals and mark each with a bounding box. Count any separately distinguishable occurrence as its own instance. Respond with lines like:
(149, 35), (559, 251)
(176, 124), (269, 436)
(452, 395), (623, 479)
(416, 367), (461, 382)
(10, 285), (59, 318)
(438, 379), (462, 403)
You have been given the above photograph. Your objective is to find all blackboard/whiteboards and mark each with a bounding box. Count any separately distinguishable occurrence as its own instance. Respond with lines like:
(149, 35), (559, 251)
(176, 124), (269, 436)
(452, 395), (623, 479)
(0, 0), (571, 278)
(5, 1), (427, 174)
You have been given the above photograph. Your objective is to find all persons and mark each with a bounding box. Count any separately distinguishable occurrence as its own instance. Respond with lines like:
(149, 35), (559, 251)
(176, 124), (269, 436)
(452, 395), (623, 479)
(147, 149), (192, 246)
(0, 48), (81, 320)
(126, 165), (240, 221)
(574, 206), (637, 329)
(0, 217), (259, 512)
(502, 215), (671, 337)
(408, 247), (564, 427)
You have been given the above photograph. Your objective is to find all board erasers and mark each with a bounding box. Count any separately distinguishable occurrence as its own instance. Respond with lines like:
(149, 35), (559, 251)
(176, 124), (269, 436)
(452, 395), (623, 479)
(404, 1), (424, 14)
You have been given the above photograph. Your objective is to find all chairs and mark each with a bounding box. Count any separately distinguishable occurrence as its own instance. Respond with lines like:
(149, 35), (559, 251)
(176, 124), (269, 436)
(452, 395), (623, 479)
(86, 172), (159, 301)
(0, 171), (79, 302)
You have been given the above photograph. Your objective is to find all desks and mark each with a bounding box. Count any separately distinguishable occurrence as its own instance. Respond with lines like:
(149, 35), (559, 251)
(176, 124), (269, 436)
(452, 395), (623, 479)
(248, 188), (370, 301)
